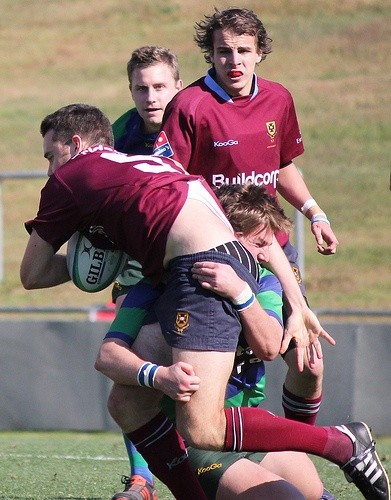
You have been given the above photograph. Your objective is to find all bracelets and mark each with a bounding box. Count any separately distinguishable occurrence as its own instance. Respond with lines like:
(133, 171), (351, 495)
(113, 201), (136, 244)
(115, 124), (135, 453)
(310, 212), (330, 226)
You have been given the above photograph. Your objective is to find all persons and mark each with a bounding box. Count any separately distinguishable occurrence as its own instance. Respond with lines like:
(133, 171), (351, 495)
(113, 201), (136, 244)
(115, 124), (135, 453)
(109, 48), (183, 500)
(95, 185), (337, 500)
(151, 7), (339, 426)
(20, 105), (391, 500)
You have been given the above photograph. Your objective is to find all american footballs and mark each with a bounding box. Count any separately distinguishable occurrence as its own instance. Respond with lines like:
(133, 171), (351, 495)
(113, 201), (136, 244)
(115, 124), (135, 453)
(66, 232), (129, 293)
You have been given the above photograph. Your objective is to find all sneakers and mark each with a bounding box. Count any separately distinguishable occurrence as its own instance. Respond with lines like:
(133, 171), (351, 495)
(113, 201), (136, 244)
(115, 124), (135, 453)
(111, 474), (158, 500)
(335, 421), (391, 500)
